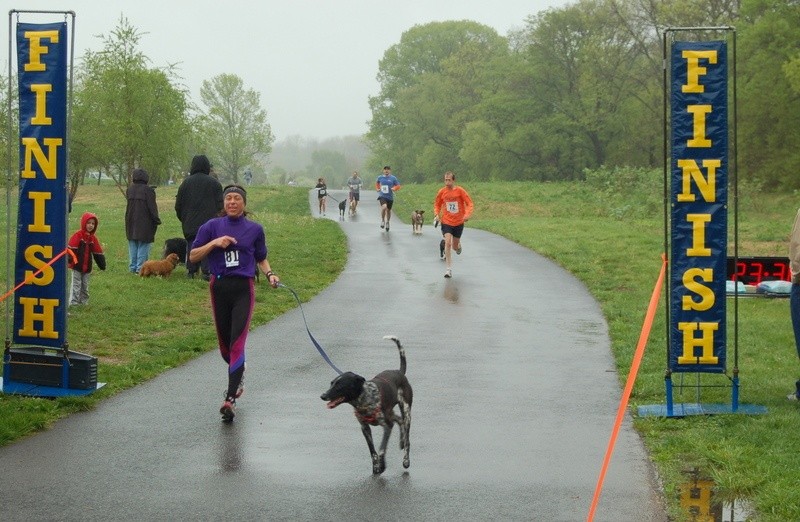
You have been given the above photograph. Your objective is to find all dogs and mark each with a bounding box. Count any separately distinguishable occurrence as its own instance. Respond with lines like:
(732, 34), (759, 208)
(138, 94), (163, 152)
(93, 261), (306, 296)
(412, 209), (425, 234)
(339, 199), (347, 216)
(349, 200), (356, 215)
(440, 240), (446, 260)
(139, 253), (180, 278)
(319, 336), (414, 475)
(162, 238), (186, 263)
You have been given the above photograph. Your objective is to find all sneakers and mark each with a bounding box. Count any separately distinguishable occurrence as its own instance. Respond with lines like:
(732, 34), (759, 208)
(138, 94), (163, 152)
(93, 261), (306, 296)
(236, 362), (247, 397)
(220, 400), (235, 423)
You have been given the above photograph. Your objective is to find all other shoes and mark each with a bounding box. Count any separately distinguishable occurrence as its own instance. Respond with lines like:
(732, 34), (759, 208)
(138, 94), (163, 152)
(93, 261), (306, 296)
(456, 240), (463, 255)
(323, 213), (326, 216)
(386, 222), (390, 231)
(380, 221), (385, 229)
(444, 270), (453, 278)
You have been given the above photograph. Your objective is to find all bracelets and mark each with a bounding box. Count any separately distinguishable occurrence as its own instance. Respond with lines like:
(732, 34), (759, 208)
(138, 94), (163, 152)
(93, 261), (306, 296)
(266, 271), (274, 281)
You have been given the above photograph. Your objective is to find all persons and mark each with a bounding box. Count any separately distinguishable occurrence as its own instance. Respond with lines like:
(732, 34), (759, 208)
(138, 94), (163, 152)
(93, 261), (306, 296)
(67, 212), (107, 307)
(787, 208), (800, 401)
(189, 184), (280, 426)
(315, 178), (328, 216)
(347, 170), (362, 215)
(376, 166), (401, 232)
(175, 156), (227, 282)
(124, 169), (162, 276)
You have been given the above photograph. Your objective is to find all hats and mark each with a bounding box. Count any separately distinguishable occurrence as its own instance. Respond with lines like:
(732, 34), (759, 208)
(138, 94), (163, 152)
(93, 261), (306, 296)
(383, 165), (390, 169)
